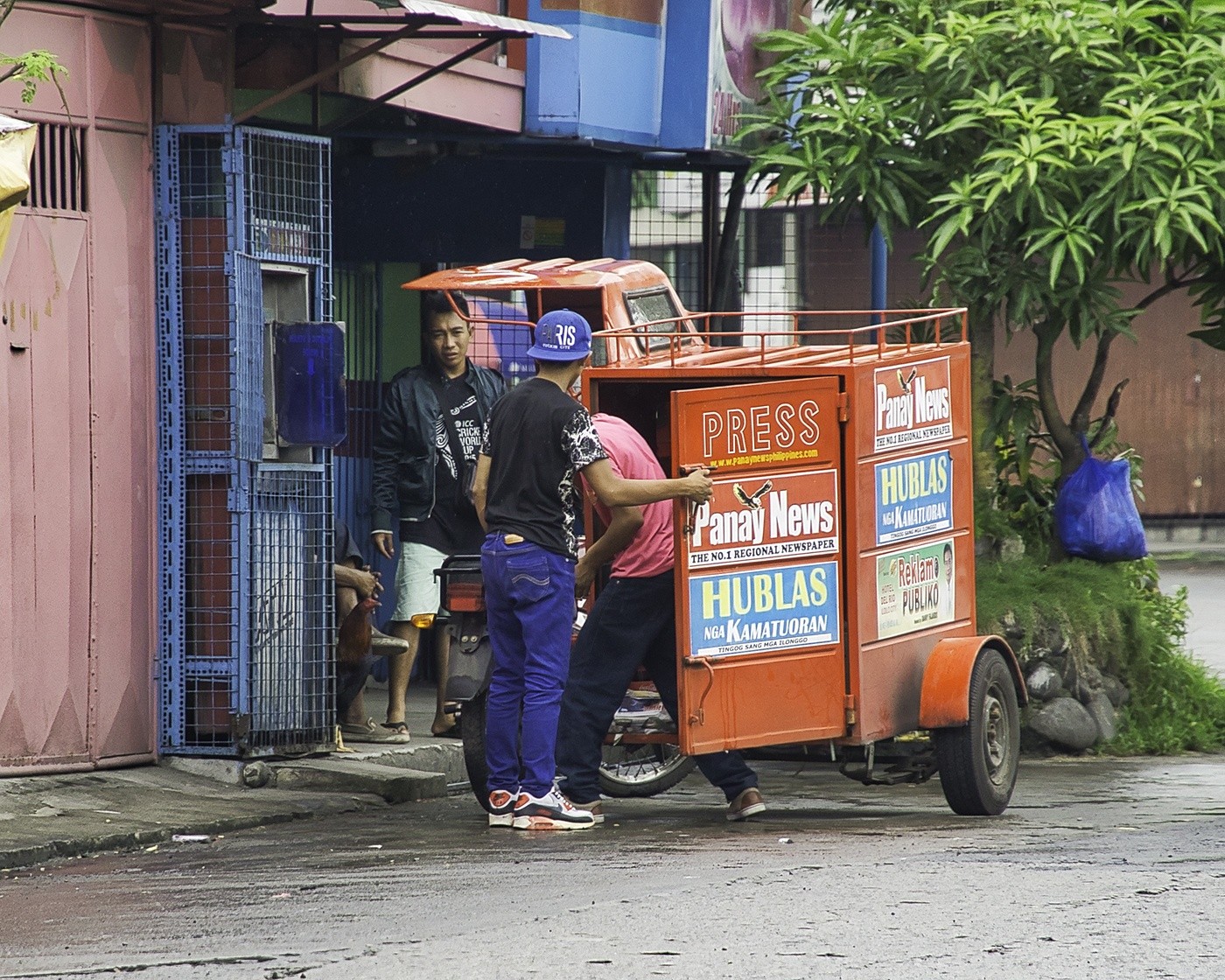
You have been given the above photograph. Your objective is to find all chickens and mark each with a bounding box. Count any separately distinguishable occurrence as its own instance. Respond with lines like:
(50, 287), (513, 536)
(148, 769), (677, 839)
(336, 597), (382, 665)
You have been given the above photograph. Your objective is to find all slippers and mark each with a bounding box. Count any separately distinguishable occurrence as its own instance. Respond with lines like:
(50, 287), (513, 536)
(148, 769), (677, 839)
(379, 722), (411, 744)
(433, 723), (462, 739)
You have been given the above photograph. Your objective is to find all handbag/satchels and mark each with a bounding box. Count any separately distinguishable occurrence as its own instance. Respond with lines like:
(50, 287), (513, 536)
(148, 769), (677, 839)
(1056, 432), (1147, 562)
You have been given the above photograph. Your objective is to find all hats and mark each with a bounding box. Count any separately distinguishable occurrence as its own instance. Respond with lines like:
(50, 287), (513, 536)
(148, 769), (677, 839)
(527, 308), (593, 361)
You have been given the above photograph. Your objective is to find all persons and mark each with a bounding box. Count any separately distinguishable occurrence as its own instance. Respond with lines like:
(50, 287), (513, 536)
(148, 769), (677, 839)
(470, 307), (713, 830)
(369, 294), (509, 740)
(315, 518), (409, 742)
(557, 413), (766, 823)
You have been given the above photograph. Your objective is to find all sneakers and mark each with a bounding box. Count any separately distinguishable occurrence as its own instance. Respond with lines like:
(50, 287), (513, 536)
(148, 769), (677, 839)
(489, 786), (520, 827)
(562, 795), (604, 824)
(727, 787), (766, 821)
(513, 785), (595, 830)
(341, 717), (395, 742)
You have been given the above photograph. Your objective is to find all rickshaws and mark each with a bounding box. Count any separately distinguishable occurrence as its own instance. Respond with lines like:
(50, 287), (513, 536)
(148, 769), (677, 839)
(402, 255), (1029, 820)
